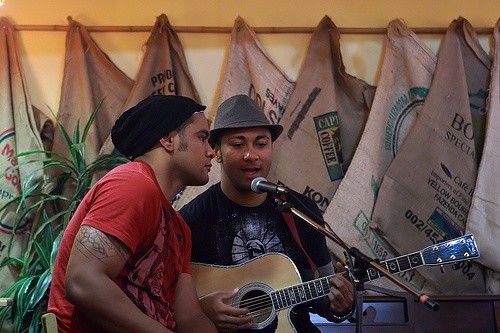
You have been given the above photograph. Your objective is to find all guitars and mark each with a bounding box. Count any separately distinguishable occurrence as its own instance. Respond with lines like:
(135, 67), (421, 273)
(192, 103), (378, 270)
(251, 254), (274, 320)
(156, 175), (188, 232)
(191, 233), (481, 333)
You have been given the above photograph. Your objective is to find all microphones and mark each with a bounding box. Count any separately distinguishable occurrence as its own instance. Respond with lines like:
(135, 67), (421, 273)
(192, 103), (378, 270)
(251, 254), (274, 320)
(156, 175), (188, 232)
(251, 177), (289, 194)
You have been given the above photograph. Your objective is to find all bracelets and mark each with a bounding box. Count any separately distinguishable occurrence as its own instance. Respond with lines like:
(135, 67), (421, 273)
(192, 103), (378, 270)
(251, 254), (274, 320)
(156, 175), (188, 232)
(333, 310), (353, 319)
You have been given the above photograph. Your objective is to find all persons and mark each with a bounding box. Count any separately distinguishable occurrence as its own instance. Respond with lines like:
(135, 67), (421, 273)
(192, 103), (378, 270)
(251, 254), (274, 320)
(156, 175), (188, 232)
(178, 94), (355, 333)
(47, 94), (218, 333)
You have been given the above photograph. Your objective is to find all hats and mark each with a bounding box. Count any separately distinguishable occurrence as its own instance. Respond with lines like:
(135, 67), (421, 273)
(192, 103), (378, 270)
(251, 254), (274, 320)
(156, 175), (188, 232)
(207, 95), (284, 150)
(111, 94), (207, 161)
